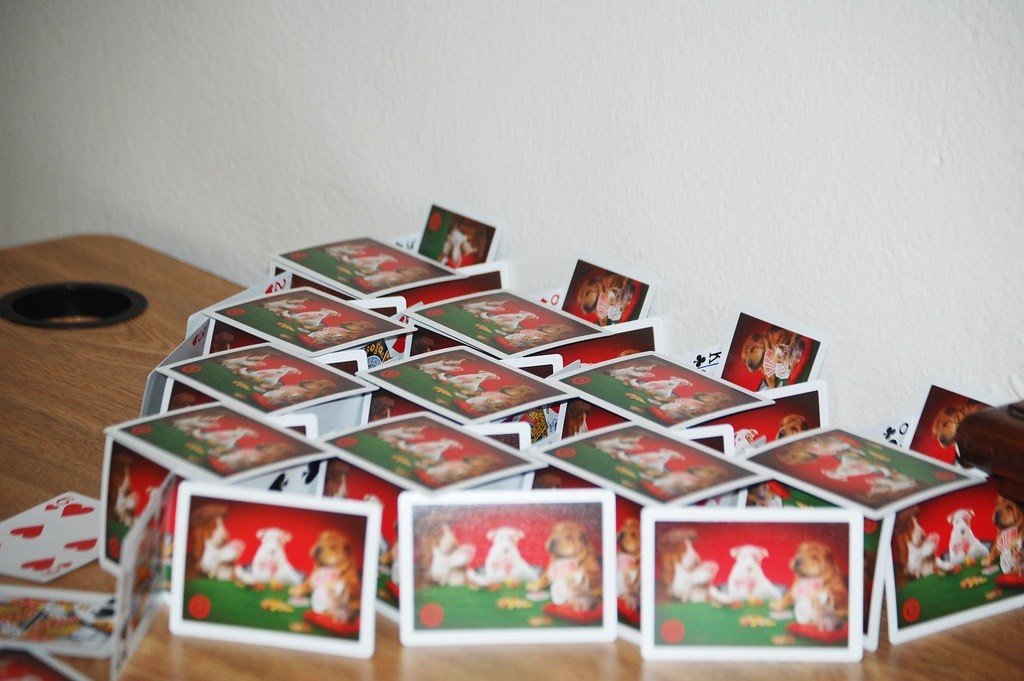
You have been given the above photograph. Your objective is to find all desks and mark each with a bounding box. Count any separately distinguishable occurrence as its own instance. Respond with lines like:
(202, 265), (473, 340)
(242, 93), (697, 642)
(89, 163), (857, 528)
(2, 230), (1018, 677)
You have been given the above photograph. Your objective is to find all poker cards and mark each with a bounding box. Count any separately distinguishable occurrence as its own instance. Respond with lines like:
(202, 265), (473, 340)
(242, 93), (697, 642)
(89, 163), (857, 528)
(0, 198), (1024, 681)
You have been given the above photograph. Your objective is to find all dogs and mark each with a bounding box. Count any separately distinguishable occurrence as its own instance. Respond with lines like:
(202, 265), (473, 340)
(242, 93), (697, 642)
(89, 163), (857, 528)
(110, 221), (1022, 633)
